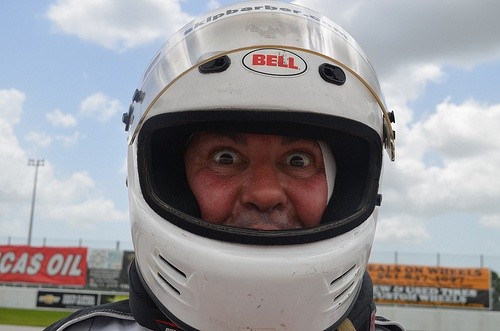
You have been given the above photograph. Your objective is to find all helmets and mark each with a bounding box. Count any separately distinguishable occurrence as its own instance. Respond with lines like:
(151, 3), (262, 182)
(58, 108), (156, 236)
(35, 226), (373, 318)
(122, 0), (396, 331)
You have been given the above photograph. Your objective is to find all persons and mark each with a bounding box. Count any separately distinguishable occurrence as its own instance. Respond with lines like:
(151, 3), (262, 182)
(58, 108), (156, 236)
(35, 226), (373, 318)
(43, 0), (406, 330)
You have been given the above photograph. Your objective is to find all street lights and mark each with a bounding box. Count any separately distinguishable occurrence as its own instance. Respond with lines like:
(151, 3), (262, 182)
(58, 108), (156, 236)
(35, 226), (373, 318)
(26, 156), (44, 247)
